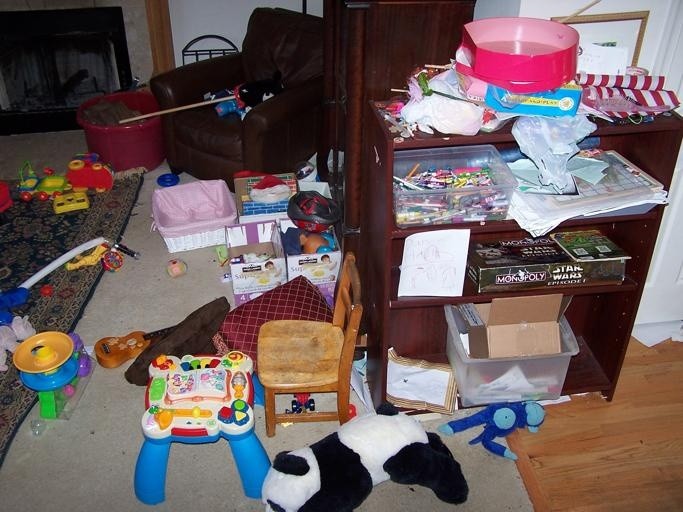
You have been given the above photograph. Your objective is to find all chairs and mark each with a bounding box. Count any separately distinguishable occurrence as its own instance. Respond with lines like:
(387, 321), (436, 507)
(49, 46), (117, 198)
(256, 253), (362, 437)
(148, 4), (327, 192)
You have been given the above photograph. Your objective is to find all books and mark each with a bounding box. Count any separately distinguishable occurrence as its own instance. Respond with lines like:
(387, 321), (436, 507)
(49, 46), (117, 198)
(549, 226), (635, 262)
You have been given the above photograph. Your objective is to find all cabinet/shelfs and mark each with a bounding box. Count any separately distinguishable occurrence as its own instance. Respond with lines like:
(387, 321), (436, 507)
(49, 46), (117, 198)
(300, 1), (683, 414)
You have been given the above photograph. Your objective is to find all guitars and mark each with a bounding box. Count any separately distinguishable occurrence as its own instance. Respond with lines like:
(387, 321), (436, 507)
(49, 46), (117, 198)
(92, 325), (174, 369)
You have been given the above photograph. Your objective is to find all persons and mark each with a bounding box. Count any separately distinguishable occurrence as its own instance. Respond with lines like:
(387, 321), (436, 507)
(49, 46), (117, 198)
(319, 255), (339, 280)
(264, 263), (286, 287)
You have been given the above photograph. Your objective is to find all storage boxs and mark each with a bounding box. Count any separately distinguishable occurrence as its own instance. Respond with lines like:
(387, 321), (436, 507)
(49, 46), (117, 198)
(280, 218), (342, 311)
(152, 180), (238, 254)
(235, 172), (299, 225)
(225, 220), (288, 306)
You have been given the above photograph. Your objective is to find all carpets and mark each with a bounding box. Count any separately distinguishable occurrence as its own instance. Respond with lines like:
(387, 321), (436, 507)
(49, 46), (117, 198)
(1, 128), (534, 511)
